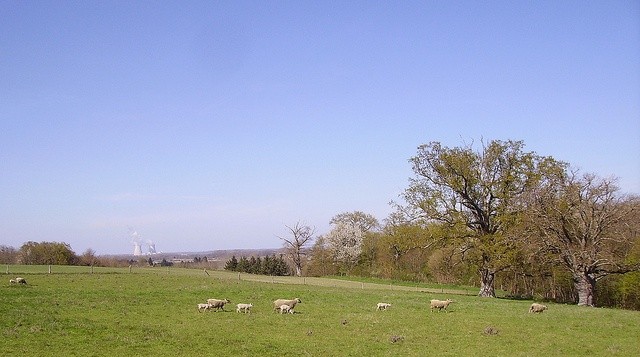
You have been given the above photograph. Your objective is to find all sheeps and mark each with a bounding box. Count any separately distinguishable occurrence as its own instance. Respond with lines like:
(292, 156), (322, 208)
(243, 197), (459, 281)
(197, 303), (214, 313)
(429, 299), (454, 314)
(279, 305), (294, 315)
(16, 278), (27, 284)
(272, 297), (302, 314)
(10, 280), (16, 284)
(236, 303), (254, 314)
(528, 303), (548, 314)
(376, 303), (391, 311)
(205, 298), (231, 313)
